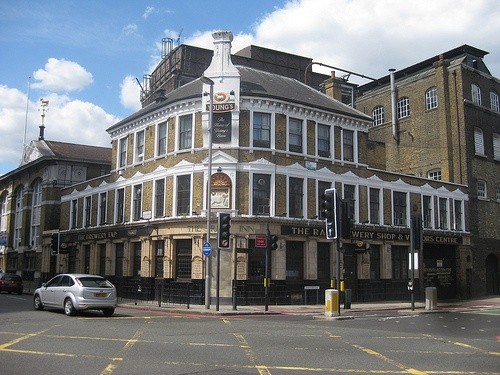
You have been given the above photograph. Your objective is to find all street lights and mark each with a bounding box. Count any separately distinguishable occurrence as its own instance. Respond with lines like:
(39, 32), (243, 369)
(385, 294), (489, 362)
(199, 74), (215, 309)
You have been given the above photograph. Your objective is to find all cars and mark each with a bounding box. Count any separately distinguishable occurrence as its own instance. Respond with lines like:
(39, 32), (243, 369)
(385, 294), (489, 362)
(0, 273), (23, 295)
(32, 273), (118, 316)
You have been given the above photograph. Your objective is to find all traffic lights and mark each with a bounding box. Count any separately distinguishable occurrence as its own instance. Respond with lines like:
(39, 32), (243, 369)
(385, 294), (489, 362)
(50, 231), (60, 256)
(269, 234), (278, 251)
(321, 188), (337, 240)
(218, 212), (231, 249)
